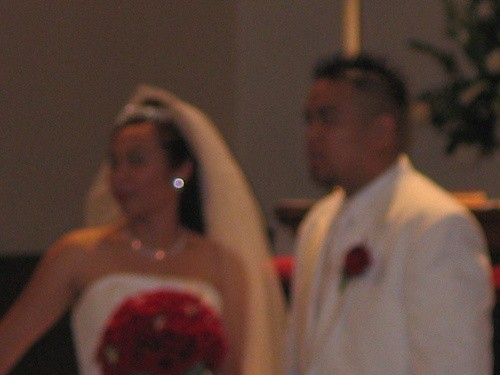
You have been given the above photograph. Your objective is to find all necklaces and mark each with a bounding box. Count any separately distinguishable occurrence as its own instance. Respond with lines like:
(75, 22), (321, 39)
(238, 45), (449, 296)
(122, 219), (189, 261)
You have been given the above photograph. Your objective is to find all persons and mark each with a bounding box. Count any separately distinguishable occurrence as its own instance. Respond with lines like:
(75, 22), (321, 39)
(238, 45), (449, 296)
(0, 82), (289, 374)
(285, 51), (493, 375)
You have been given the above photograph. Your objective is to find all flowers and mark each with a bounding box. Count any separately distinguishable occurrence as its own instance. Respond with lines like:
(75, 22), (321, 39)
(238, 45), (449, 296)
(96, 289), (228, 375)
(337, 245), (373, 291)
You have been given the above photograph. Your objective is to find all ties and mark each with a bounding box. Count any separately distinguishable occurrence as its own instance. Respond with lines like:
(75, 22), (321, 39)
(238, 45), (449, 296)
(308, 201), (352, 345)
